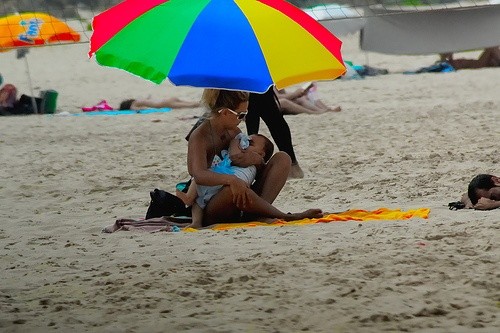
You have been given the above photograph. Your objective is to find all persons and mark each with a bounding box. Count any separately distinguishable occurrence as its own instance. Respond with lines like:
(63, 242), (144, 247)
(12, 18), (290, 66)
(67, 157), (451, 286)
(461, 174), (500, 210)
(183, 87), (324, 230)
(343, 61), (389, 76)
(0, 75), (17, 114)
(176, 134), (274, 230)
(113, 96), (203, 110)
(435, 46), (500, 69)
(245, 82), (342, 178)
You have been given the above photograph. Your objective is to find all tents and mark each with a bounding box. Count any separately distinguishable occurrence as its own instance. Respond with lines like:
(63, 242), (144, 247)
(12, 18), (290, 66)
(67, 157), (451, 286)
(359, 4), (500, 73)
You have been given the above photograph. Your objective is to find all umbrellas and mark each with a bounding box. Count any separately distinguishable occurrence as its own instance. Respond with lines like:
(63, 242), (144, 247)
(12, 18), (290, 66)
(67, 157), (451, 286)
(301, 3), (367, 37)
(0, 11), (80, 113)
(88, 0), (346, 94)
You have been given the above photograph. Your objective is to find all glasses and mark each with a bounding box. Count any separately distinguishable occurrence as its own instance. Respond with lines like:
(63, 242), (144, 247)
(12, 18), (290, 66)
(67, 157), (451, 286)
(217, 106), (248, 121)
